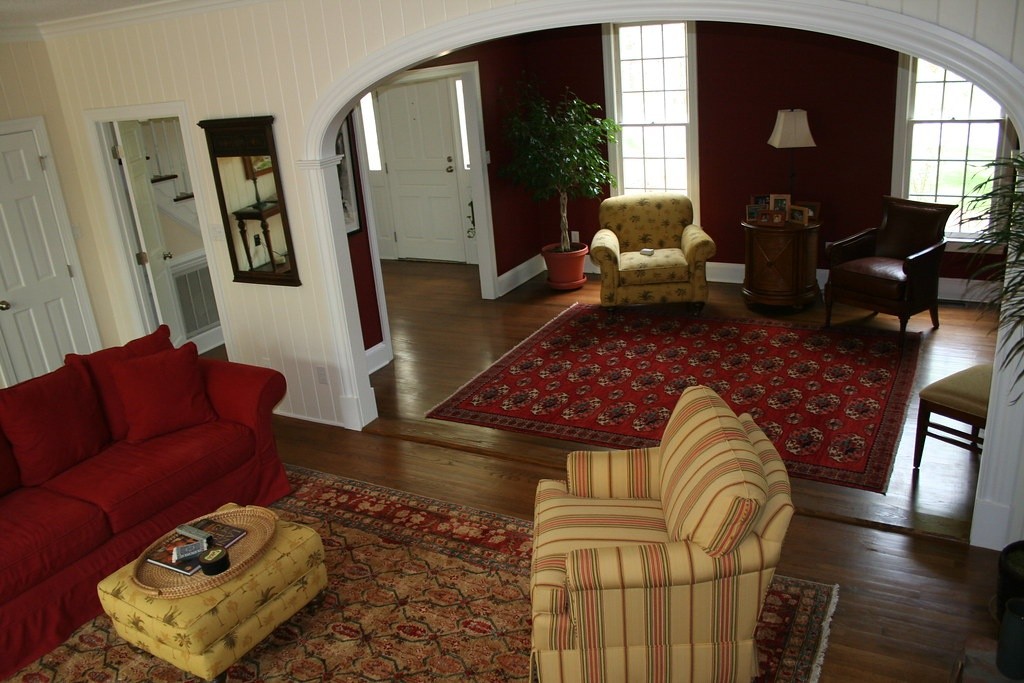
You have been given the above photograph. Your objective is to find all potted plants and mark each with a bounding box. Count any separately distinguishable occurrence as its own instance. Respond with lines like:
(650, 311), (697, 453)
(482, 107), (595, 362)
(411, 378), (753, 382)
(502, 80), (623, 289)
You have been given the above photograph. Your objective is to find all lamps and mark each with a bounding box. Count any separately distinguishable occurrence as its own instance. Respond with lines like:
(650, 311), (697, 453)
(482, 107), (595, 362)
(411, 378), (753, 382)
(766, 106), (818, 202)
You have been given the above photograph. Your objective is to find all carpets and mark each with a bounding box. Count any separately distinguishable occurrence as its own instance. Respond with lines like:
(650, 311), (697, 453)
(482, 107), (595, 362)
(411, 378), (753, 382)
(424, 302), (924, 496)
(9, 462), (840, 683)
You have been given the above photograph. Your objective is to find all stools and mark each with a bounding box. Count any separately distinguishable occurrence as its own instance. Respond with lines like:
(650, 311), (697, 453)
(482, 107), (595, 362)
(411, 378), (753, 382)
(97, 502), (330, 683)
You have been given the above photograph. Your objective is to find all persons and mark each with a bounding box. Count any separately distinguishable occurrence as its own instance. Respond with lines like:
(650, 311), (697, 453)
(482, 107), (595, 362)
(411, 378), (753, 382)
(774, 216), (779, 223)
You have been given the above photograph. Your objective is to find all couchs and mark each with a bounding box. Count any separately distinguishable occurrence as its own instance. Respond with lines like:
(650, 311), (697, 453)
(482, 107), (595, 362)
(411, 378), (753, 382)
(591, 196), (718, 315)
(527, 385), (796, 683)
(0, 322), (290, 683)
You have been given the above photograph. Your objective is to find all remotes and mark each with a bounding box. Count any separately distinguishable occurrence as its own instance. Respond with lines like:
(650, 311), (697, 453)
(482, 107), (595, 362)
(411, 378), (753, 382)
(175, 525), (213, 543)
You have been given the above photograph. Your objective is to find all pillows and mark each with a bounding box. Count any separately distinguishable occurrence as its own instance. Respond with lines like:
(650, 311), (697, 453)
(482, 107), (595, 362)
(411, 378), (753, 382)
(107, 340), (218, 445)
(64, 324), (175, 443)
(0, 358), (109, 488)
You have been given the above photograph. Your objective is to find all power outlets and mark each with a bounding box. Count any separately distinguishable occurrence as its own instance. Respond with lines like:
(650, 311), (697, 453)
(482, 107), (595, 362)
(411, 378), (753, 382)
(571, 231), (580, 244)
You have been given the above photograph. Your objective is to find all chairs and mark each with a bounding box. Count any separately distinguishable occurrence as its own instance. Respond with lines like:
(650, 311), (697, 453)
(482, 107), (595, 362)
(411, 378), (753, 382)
(822, 195), (958, 342)
(914, 362), (992, 469)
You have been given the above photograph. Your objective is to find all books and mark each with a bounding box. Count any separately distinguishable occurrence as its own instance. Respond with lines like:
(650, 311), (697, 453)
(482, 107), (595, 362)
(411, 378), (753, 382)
(194, 518), (246, 549)
(147, 535), (201, 575)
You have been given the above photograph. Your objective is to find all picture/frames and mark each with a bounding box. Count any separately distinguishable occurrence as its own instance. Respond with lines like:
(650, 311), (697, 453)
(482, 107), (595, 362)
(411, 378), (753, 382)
(787, 205), (809, 226)
(770, 194), (791, 221)
(758, 209), (785, 227)
(751, 193), (770, 208)
(746, 204), (768, 222)
